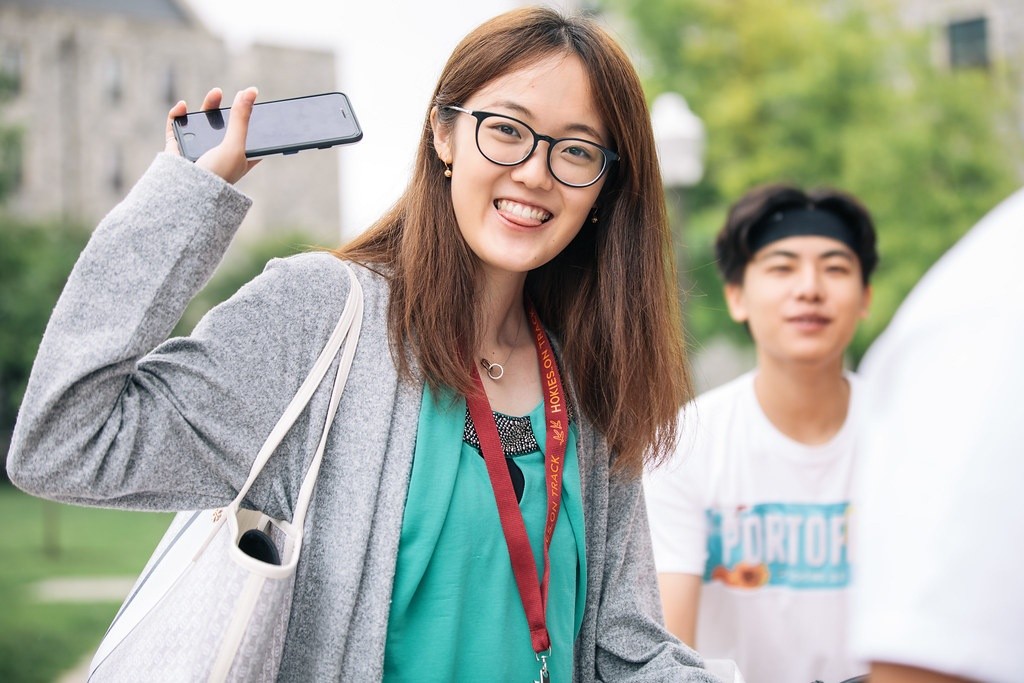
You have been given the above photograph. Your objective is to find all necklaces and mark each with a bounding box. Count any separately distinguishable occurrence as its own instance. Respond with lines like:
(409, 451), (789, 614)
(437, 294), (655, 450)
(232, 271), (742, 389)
(475, 311), (528, 380)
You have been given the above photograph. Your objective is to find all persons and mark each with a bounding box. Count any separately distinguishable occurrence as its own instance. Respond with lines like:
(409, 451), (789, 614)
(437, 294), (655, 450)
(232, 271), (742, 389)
(5, 5), (733, 683)
(841, 182), (1024, 683)
(641, 178), (880, 683)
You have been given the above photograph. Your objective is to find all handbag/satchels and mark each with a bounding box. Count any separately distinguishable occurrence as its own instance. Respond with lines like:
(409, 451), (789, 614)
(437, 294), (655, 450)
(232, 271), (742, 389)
(86, 256), (363, 683)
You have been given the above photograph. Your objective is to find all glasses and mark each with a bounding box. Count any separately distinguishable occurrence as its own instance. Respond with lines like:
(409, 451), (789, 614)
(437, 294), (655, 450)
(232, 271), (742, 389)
(446, 105), (620, 188)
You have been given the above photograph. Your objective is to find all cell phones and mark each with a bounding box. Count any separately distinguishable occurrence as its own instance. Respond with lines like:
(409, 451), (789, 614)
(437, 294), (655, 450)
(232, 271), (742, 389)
(172, 93), (363, 162)
